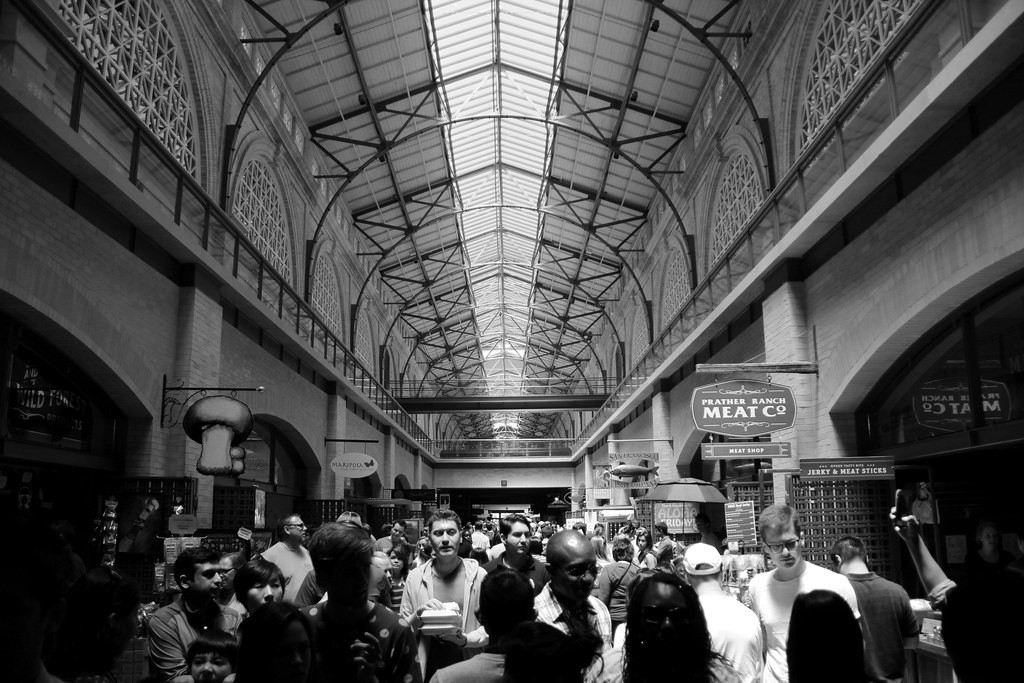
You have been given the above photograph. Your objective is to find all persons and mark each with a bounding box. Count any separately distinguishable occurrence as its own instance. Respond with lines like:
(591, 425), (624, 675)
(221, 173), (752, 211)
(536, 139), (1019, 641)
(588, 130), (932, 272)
(33, 489), (1024, 683)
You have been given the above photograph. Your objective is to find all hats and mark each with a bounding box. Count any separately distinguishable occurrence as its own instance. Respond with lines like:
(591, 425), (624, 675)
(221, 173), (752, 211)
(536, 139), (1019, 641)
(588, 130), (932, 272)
(682, 543), (723, 576)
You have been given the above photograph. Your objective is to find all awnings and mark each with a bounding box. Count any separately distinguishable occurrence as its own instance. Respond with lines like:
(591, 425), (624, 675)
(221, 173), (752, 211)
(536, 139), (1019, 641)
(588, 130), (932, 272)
(353, 471), (381, 498)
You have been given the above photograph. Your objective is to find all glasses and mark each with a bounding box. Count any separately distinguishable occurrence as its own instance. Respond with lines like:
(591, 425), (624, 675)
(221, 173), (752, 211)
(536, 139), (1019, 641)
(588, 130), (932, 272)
(218, 567), (234, 574)
(286, 523), (306, 528)
(639, 603), (691, 627)
(550, 561), (602, 577)
(762, 539), (800, 554)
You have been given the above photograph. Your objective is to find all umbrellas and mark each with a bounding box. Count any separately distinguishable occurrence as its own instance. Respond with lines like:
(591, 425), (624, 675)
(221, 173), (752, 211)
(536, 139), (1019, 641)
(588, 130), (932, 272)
(640, 478), (727, 543)
